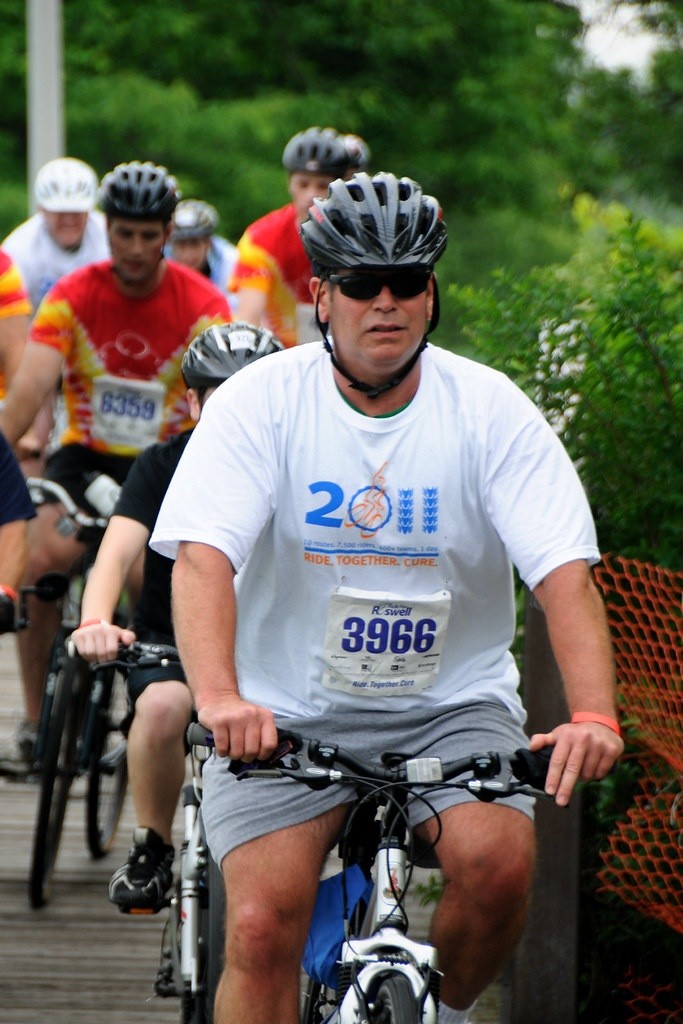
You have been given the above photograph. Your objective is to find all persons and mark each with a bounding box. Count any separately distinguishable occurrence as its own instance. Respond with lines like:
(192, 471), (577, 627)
(71, 319), (288, 905)
(0, 159), (238, 779)
(149, 172), (623, 1024)
(225, 126), (370, 349)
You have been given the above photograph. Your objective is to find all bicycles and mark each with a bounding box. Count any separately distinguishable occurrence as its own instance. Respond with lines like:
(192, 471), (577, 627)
(184, 718), (619, 1024)
(14, 473), (157, 910)
(68, 635), (249, 995)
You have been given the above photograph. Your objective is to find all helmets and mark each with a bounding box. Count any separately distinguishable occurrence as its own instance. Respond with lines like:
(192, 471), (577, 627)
(299, 169), (454, 275)
(167, 197), (223, 242)
(280, 132), (374, 178)
(179, 321), (287, 394)
(93, 159), (184, 224)
(31, 154), (101, 214)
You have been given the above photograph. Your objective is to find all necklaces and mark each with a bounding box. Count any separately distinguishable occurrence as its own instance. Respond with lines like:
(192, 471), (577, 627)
(337, 387), (413, 418)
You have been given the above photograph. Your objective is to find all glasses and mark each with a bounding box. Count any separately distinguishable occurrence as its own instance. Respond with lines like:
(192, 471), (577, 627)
(315, 265), (433, 300)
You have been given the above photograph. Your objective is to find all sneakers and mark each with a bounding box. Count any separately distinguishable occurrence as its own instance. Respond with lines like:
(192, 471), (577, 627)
(44, 671), (81, 697)
(0, 720), (50, 781)
(196, 856), (214, 910)
(107, 824), (176, 910)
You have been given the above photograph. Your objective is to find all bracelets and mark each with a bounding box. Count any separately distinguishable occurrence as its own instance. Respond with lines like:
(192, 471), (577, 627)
(572, 712), (621, 737)
(0, 584), (16, 600)
(79, 619), (107, 627)
